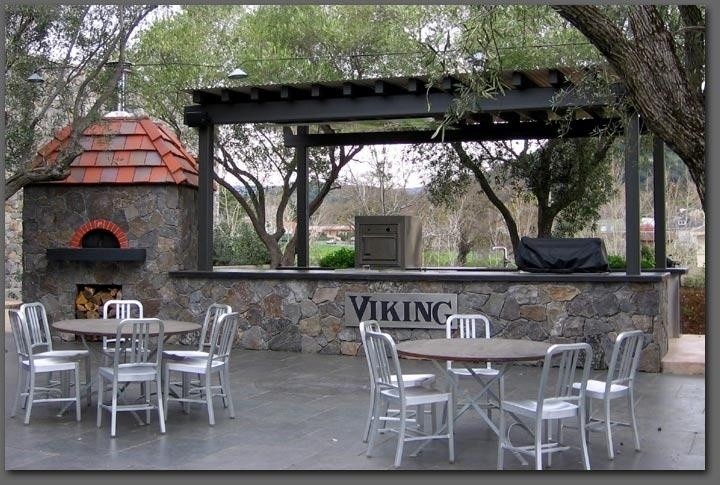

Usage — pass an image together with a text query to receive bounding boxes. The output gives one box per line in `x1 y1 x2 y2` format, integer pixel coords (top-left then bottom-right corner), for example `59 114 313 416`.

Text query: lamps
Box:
228 68 248 79
468 51 488 62
27 71 44 82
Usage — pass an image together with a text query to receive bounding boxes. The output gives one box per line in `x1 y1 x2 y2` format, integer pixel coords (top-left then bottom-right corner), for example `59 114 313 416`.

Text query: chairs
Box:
8 300 239 439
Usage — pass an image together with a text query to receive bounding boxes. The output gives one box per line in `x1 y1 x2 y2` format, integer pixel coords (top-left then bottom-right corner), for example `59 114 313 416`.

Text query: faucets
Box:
491 245 511 268
